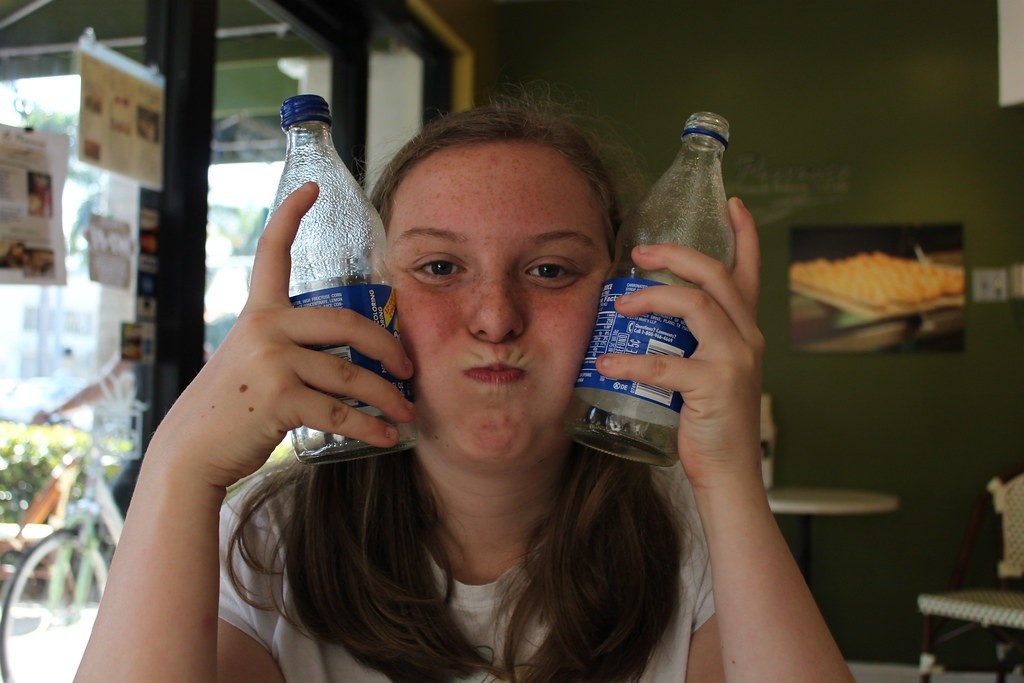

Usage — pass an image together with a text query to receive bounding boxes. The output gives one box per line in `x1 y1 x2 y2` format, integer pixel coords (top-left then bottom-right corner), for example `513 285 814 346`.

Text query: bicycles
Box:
0 392 122 683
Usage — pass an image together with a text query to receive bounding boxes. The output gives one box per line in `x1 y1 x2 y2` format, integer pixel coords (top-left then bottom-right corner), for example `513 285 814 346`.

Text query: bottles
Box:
759 394 777 495
562 111 738 469
267 93 419 464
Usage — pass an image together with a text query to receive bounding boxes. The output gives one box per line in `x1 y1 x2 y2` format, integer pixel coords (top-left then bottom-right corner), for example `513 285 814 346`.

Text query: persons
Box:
31 341 210 518
75 111 853 683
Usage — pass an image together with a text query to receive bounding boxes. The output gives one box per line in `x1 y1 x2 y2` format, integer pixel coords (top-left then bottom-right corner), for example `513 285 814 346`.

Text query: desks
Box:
766 487 902 585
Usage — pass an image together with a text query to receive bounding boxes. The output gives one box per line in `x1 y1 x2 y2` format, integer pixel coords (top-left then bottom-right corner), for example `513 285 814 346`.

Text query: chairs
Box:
917 474 1024 683
